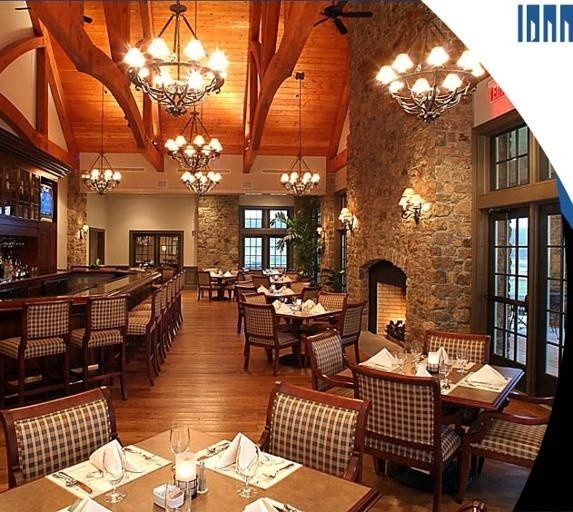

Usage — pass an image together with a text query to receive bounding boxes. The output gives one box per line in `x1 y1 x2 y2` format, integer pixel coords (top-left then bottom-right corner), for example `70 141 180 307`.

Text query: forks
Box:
263 463 295 478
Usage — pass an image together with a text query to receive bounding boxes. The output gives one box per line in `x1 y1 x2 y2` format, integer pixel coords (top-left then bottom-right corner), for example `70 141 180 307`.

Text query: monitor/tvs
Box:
39 184 55 220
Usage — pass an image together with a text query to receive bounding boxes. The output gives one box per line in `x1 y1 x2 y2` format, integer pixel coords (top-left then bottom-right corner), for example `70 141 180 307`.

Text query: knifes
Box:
59 472 94 493
197 442 231 461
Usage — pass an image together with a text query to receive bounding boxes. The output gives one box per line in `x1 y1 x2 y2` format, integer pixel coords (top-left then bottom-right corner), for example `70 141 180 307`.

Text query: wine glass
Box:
235 435 260 498
165 481 191 512
169 426 190 469
439 357 453 390
103 449 127 502
277 296 317 316
395 349 408 374
458 347 470 373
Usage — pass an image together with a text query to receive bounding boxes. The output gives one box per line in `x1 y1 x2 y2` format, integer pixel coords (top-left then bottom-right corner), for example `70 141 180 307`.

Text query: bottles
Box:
196 462 209 495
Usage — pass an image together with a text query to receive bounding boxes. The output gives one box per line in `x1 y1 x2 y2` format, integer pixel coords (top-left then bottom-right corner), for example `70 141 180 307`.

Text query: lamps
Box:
399 187 431 225
279 73 320 198
338 208 356 234
165 107 223 173
79 81 121 198
376 19 483 124
122 0 226 118
179 170 220 198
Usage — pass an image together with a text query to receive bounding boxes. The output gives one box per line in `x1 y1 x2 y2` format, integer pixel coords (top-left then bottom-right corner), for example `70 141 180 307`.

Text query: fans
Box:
314 0 374 34
